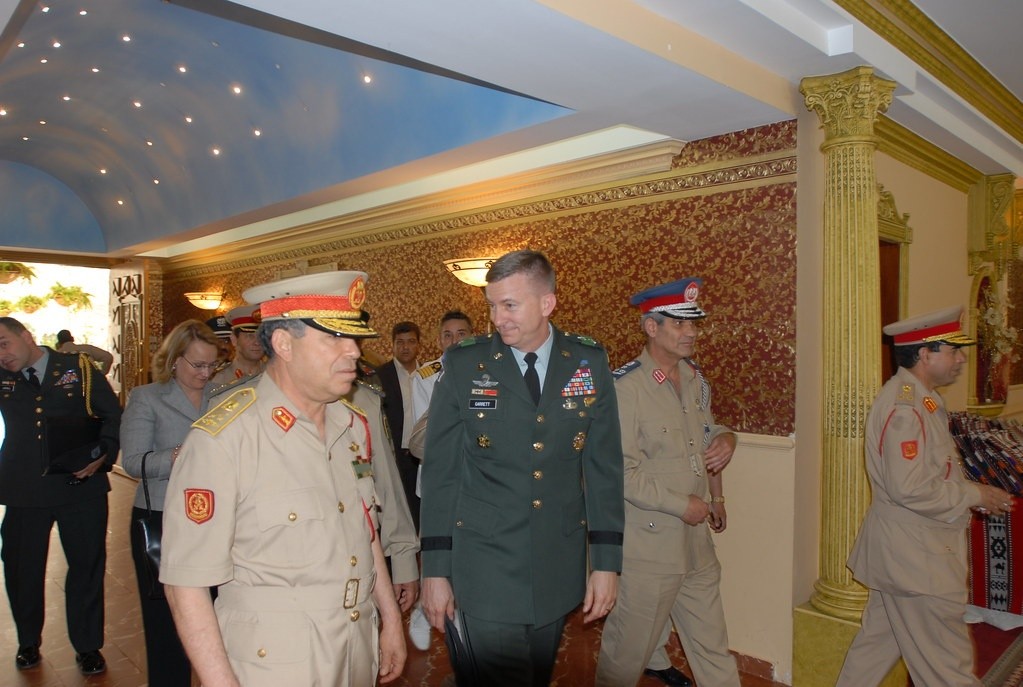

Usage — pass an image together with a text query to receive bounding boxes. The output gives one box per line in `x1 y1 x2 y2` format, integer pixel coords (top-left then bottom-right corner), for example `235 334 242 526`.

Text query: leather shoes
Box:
644 666 692 687
17 645 41 669
76 648 106 674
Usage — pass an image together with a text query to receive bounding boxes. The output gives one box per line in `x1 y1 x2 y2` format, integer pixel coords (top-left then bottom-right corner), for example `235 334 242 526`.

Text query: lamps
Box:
184 292 223 318
442 256 499 337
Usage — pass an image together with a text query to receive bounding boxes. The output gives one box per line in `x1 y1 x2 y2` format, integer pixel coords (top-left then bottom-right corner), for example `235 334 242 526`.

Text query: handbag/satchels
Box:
42 414 104 473
137 450 165 599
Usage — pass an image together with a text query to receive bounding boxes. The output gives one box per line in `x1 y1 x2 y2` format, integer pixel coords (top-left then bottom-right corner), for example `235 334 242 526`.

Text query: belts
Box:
399 449 413 456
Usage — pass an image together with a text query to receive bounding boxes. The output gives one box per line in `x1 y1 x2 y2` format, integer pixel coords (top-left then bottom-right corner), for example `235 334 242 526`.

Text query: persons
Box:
418 250 625 687
55 330 113 375
594 276 742 687
158 271 407 687
835 306 1013 687
206 304 475 649
120 319 230 687
0 317 122 674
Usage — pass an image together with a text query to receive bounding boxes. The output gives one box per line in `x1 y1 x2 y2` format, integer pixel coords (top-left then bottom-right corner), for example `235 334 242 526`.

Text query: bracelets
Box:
712 496 724 503
174 443 182 459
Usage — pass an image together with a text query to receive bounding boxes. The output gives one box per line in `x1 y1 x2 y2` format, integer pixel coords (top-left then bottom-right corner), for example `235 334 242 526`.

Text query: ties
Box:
523 352 541 407
26 368 40 392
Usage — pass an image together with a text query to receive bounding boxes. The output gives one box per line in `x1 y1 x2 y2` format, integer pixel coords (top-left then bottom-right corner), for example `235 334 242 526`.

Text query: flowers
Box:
976 284 1023 362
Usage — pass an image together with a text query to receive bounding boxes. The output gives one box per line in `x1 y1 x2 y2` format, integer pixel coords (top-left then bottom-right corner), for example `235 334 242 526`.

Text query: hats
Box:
630 278 708 320
883 305 977 346
445 608 482 687
53 441 103 471
205 316 233 338
242 270 377 338
358 310 370 324
225 303 261 332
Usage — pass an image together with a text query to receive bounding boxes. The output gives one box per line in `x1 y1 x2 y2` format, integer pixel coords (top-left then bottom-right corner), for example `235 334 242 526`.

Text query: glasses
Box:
180 354 219 369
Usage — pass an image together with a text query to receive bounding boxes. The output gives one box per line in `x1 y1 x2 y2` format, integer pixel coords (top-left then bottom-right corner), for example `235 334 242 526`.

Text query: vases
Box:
983 355 1008 405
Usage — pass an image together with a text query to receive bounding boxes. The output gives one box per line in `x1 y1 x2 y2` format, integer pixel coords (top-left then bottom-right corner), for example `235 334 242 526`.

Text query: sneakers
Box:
406 606 432 651
